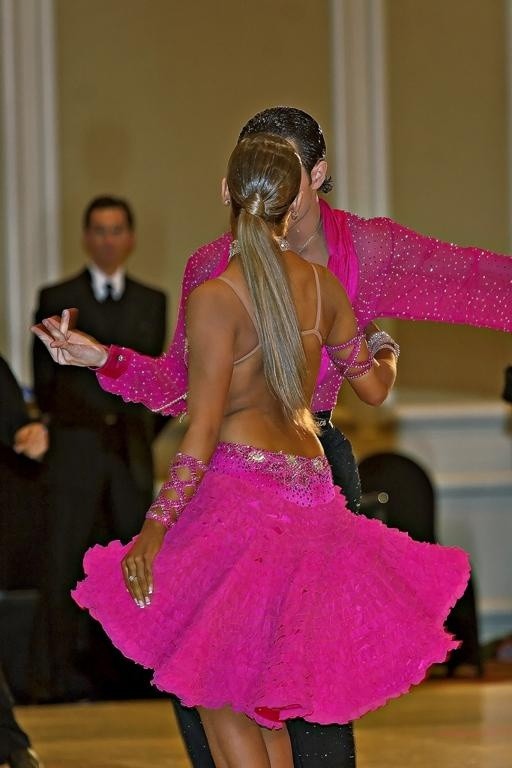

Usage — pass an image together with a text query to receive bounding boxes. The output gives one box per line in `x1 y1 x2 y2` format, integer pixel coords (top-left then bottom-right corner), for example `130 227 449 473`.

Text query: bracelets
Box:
143 450 207 533
366 331 399 359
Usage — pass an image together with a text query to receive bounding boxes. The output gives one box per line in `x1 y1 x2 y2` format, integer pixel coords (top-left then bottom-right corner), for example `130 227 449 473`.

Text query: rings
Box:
126 574 137 582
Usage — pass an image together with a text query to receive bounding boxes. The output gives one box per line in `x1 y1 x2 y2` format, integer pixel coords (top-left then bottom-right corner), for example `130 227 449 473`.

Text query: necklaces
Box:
227 235 290 256
294 214 324 255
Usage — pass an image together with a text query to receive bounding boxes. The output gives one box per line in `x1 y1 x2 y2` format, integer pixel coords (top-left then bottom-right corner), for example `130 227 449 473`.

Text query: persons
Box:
28 192 169 704
69 132 470 767
0 361 52 705
27 104 512 767
